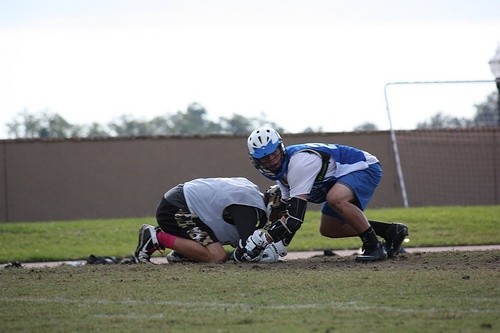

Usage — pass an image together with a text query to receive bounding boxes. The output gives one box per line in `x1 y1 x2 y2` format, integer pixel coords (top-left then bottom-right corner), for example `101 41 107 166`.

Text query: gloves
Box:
259 238 288 263
232 228 272 263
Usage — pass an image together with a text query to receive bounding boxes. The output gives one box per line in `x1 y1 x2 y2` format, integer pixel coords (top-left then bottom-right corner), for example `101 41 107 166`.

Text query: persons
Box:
241 127 409 262
135 176 294 264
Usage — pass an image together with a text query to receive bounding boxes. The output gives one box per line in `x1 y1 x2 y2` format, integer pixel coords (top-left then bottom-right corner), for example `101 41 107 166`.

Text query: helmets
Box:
246 126 288 181
264 184 285 221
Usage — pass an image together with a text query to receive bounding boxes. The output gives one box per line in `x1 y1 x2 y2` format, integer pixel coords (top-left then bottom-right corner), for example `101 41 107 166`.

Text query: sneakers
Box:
355 240 387 263
383 221 408 257
167 249 189 265
135 225 165 265
